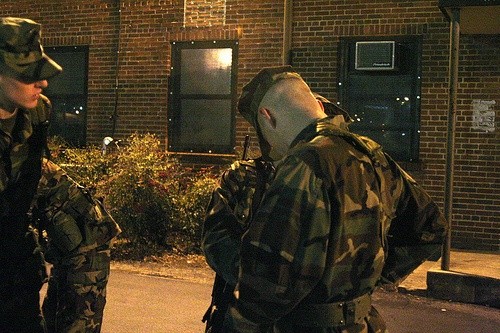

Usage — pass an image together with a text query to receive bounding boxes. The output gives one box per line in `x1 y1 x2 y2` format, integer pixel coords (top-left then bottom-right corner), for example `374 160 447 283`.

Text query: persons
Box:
220 64 448 333
0 16 61 333
202 94 354 332
29 156 123 333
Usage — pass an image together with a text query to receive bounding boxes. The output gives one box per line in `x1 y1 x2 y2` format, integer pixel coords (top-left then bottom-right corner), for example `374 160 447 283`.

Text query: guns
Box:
202 135 251 333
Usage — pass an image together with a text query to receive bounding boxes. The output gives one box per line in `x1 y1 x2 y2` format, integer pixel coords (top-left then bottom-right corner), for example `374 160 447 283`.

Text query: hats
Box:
0 16 63 84
237 65 301 161
